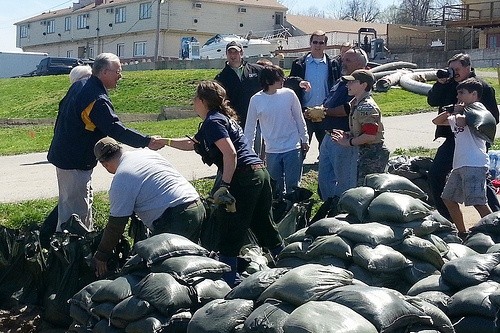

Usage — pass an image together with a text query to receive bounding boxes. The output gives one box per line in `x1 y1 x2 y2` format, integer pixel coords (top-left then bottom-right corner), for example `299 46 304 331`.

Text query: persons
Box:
304 48 369 204
153 80 285 256
427 53 499 224
214 41 267 162
92 136 206 277
286 30 340 162
431 77 493 233
69 64 94 85
243 64 310 195
47 52 165 233
331 69 390 187
329 42 353 66
256 59 273 67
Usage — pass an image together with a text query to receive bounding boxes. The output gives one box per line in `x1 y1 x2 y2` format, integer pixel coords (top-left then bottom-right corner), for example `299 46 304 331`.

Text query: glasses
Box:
352 45 357 53
104 67 122 74
312 40 325 45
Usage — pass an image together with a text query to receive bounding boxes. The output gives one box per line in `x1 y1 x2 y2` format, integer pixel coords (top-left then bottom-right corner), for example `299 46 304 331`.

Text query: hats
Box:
226 41 243 52
341 69 376 87
94 137 118 160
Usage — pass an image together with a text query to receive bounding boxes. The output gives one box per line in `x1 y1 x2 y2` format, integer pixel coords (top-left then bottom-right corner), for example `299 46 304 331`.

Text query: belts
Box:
237 164 265 172
325 129 333 134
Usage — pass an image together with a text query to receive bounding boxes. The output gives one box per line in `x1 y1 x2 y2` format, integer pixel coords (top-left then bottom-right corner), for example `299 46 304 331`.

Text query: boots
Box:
221 257 237 286
268 241 286 267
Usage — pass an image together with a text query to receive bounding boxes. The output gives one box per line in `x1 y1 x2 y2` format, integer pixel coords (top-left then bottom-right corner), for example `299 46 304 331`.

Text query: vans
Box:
35 58 93 77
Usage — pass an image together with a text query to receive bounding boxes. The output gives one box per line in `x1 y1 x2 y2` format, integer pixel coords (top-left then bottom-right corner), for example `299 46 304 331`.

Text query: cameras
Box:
436 67 455 79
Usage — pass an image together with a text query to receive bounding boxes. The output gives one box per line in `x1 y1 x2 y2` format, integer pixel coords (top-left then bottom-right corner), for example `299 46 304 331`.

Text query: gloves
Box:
214 187 228 206
226 192 236 213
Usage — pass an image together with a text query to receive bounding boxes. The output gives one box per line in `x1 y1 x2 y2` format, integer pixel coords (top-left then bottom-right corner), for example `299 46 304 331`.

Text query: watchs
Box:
167 138 172 146
220 180 230 187
349 138 353 146
